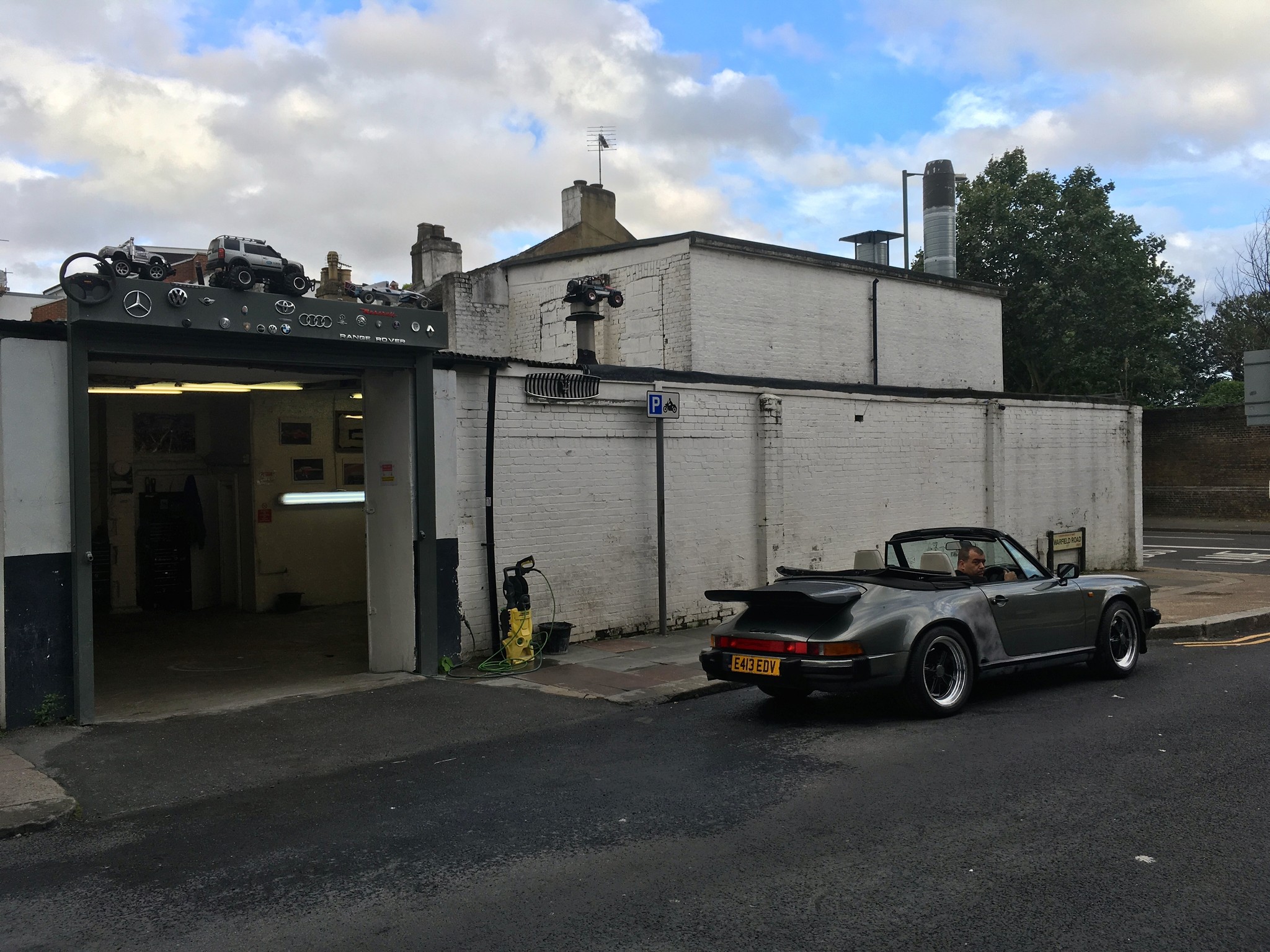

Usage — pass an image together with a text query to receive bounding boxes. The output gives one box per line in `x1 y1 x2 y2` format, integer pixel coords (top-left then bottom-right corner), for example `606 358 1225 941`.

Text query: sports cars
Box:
698 526 1163 718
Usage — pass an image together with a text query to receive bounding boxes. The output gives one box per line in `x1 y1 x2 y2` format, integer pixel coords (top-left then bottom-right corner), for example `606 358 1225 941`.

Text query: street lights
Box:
901 169 969 270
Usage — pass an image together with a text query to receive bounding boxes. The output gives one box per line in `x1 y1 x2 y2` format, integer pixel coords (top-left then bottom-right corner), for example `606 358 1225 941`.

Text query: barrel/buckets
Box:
538 622 576 655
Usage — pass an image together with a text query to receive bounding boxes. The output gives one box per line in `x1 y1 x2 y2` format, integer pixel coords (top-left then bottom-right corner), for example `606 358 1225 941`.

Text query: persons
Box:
955 545 1018 582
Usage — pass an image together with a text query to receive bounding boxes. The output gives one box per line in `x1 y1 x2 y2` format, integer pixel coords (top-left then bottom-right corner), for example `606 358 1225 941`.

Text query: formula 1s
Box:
345 280 432 311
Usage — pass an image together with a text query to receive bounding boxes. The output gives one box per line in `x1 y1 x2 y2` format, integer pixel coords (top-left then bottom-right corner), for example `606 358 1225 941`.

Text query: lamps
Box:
999 403 1006 411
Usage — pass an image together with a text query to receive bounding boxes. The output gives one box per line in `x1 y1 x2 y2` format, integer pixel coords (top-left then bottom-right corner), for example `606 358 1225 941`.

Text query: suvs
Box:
204 235 317 296
565 275 624 308
97 238 176 280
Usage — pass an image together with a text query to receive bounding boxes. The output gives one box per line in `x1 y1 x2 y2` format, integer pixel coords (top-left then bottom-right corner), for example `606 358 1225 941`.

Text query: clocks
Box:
109 460 134 493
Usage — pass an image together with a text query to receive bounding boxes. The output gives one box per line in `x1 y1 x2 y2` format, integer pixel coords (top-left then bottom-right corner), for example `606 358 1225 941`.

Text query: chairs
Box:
919 551 957 576
853 550 886 569
